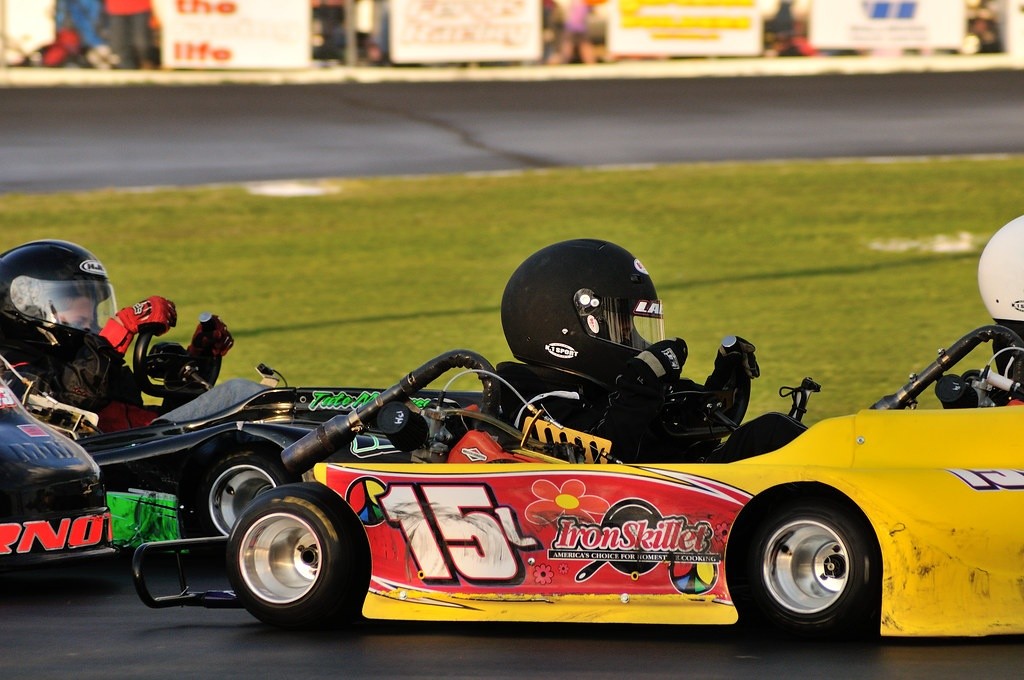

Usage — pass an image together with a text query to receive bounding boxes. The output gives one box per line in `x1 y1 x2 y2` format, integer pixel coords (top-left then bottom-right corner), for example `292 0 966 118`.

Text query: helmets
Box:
502 238 663 390
0 240 117 408
977 212 1023 322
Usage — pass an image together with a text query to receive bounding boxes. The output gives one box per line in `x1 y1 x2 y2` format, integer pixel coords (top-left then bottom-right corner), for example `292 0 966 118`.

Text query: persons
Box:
311 0 388 67
495 238 760 464
541 0 606 65
968 10 1000 54
975 216 1023 405
1 239 234 441
764 31 815 57
28 1 159 69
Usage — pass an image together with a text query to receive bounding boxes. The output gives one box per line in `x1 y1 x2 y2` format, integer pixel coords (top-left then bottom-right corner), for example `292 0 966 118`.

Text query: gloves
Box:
623 337 687 390
706 336 760 397
115 296 177 337
187 314 234 362
